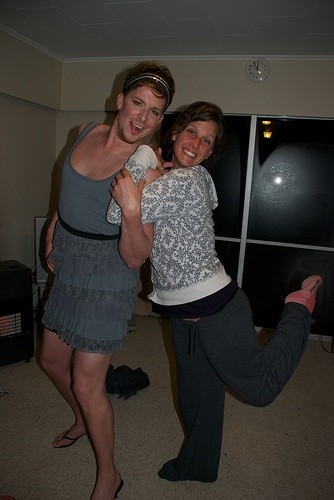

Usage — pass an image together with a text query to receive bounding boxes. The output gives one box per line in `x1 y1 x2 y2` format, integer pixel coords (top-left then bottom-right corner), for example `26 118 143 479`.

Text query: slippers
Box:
88 478 125 500
52 425 91 449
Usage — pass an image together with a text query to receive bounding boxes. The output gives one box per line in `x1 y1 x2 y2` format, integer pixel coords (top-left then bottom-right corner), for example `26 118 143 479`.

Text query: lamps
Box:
263 129 273 139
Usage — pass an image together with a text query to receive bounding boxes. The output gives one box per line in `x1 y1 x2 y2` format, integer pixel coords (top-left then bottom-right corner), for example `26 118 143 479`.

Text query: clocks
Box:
245 57 271 83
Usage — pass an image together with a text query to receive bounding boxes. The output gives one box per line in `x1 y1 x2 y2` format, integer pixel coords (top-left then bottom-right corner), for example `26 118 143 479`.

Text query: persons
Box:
38 63 176 500
104 102 323 483
